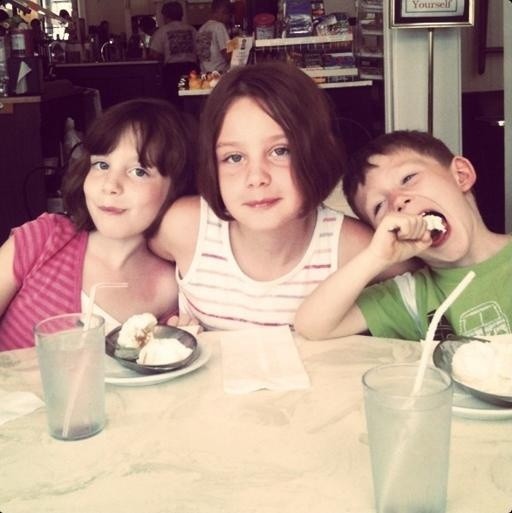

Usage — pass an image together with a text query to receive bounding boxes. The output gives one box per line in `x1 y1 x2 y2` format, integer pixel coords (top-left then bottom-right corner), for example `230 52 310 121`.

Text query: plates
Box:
105 323 197 375
419 339 512 423
432 333 512 410
104 331 210 387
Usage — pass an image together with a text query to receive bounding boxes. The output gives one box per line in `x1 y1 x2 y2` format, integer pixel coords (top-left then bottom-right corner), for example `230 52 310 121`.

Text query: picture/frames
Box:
389 0 475 30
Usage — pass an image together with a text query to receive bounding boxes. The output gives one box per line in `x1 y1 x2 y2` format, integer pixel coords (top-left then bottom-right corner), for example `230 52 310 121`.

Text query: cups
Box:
33 311 107 441
361 361 455 513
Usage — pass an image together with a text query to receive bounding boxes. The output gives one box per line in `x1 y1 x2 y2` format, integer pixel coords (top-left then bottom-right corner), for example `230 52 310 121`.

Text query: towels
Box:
0 388 45 425
220 325 311 394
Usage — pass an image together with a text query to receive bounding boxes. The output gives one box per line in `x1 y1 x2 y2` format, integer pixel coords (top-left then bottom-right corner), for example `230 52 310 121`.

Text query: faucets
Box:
138 39 148 61
100 37 116 62
48 41 64 67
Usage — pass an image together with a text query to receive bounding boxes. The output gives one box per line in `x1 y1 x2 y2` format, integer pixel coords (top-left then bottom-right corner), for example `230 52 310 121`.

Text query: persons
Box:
293 128 512 344
145 58 422 336
25 0 234 114
0 96 195 353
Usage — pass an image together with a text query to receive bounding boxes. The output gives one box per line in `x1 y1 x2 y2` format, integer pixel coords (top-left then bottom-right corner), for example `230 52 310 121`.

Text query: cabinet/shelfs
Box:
354 0 384 80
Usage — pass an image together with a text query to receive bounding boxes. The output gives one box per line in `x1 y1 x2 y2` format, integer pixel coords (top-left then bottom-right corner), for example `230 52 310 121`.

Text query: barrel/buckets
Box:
253 13 276 40
